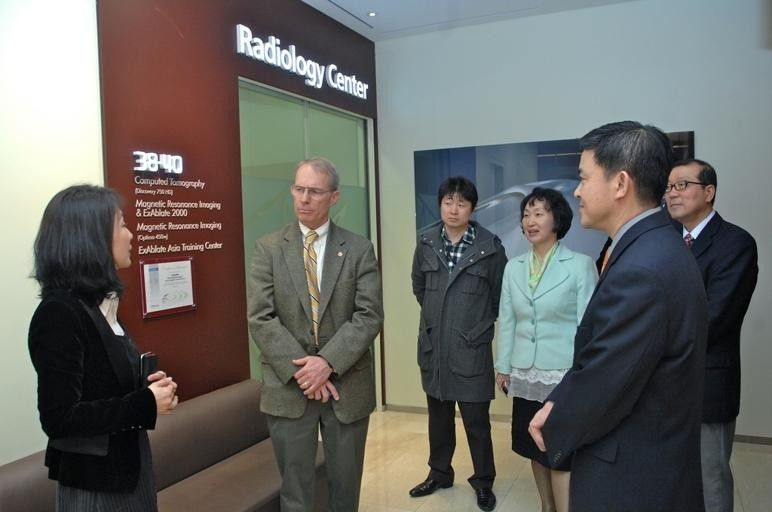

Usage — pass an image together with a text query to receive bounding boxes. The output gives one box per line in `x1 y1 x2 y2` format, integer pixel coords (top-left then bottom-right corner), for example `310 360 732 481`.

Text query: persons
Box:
495 186 600 512
665 159 759 512
528 120 706 512
242 155 385 512
410 174 509 512
26 182 178 512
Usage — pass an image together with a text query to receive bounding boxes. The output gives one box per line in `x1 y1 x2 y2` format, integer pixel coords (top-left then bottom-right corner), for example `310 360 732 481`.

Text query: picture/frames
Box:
139 256 199 322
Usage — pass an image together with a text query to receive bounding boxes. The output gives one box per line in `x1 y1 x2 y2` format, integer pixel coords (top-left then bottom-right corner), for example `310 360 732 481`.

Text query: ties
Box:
680 235 694 251
300 232 323 347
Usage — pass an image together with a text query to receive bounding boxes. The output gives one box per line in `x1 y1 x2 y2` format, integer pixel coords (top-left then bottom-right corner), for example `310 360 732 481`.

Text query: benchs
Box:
0 379 328 512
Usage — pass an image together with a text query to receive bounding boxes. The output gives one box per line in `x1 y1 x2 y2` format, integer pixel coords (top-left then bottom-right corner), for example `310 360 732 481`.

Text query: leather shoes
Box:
409 477 455 498
477 487 498 512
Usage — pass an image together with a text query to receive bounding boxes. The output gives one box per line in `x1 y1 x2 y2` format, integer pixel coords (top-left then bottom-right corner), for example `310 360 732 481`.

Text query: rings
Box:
304 382 309 387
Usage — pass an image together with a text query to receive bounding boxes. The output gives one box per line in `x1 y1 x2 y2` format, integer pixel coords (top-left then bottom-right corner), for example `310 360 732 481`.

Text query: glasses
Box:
666 179 707 192
290 184 330 197
